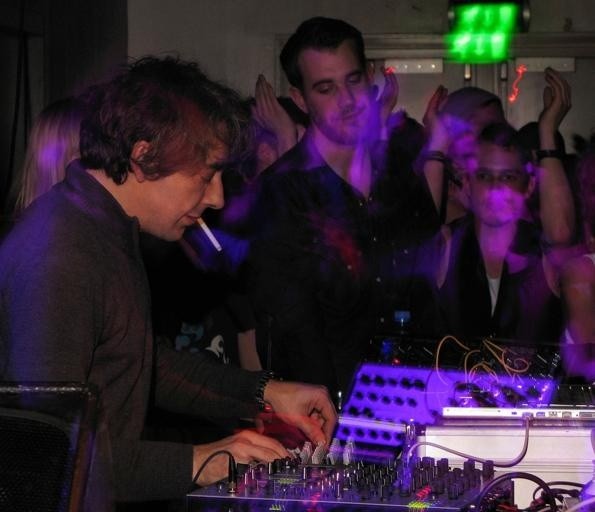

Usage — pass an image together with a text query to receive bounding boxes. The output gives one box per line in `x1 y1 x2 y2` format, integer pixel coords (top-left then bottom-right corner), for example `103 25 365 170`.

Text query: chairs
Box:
0 381 101 512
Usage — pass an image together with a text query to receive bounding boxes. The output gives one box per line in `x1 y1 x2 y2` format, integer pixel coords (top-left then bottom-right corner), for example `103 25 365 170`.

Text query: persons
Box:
1 54 337 512
4 99 86 237
153 17 594 394
